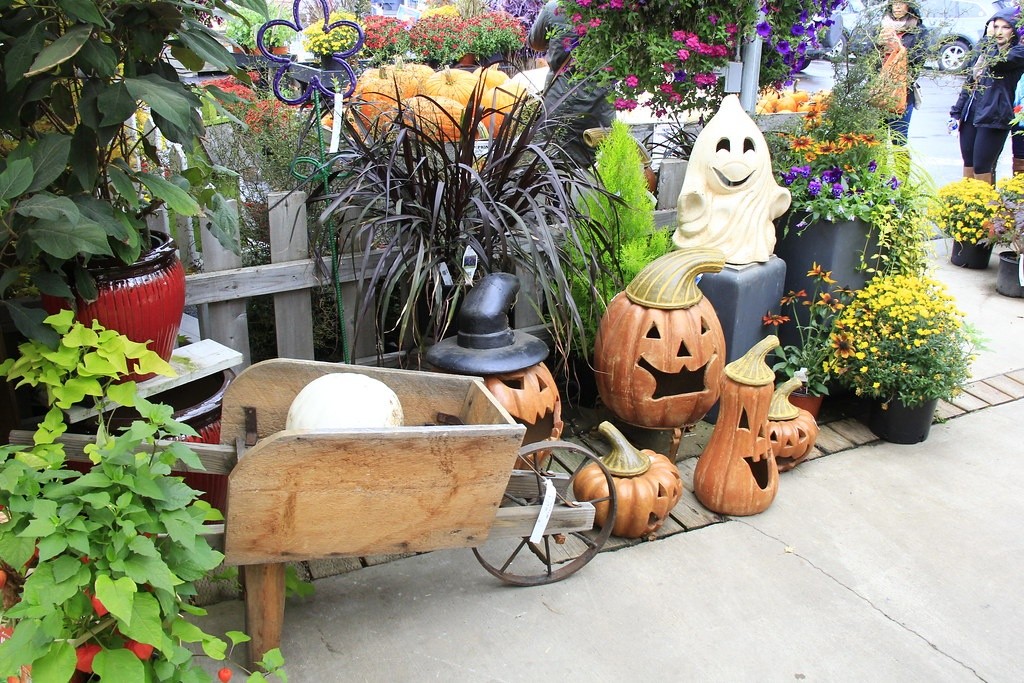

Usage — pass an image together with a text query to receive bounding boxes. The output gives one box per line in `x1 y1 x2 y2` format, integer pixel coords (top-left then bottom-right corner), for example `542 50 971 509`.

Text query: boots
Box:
974 173 994 192
963 166 973 181
1012 157 1024 177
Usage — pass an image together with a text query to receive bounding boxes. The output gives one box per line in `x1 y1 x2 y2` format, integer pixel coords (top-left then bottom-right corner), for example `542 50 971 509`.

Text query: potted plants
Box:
257 58 675 416
0 0 271 385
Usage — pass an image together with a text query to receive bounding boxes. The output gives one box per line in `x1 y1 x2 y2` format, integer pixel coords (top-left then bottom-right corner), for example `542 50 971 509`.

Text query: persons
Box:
863 0 930 147
946 7 1024 186
1011 73 1024 178
525 0 616 168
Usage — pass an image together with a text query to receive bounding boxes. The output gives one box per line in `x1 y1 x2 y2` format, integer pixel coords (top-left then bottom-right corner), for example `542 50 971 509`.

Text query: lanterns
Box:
766 377 817 472
593 246 726 428
573 421 682 537
426 273 563 470
694 335 779 516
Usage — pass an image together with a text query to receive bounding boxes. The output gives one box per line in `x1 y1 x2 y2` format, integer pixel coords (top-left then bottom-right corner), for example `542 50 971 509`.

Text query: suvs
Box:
823 1 1001 72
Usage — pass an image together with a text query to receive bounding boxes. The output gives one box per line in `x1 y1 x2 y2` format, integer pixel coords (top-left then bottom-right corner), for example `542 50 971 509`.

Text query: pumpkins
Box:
753 78 831 112
286 372 404 432
324 54 550 142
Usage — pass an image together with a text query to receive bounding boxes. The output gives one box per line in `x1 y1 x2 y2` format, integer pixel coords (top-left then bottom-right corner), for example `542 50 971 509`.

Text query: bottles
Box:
948 119 959 136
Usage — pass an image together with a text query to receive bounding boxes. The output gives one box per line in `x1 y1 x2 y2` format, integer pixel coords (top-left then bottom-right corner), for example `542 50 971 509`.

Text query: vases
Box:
233 45 246 54
788 392 825 417
697 255 787 425
867 393 939 445
996 251 1024 298
85 368 235 526
429 58 442 69
457 53 474 67
271 46 287 55
952 239 994 269
321 53 345 70
773 207 885 357
253 47 269 57
478 48 503 67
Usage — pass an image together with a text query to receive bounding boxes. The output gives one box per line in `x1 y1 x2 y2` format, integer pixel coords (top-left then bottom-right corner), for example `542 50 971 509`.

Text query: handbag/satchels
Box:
911 81 923 110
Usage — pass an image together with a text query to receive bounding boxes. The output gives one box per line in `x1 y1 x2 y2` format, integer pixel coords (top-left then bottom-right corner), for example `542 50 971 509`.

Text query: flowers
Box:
546 0 1024 410
141 0 529 180
0 308 315 683
